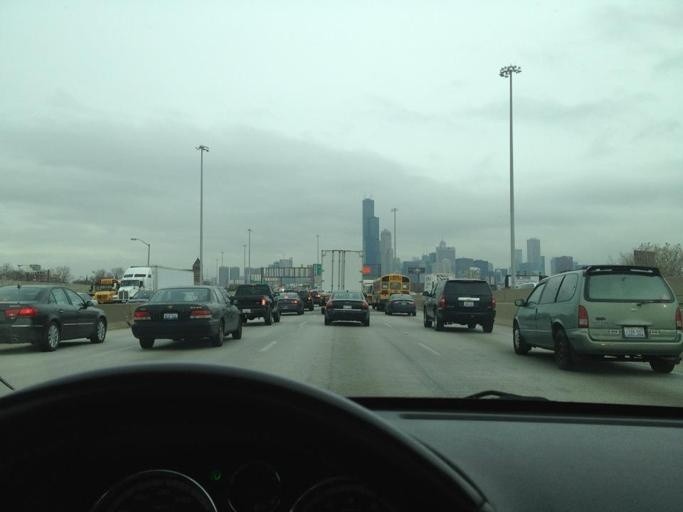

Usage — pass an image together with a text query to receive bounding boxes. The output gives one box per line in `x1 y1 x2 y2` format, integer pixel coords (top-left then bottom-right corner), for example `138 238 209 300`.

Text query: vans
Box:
512 265 682 372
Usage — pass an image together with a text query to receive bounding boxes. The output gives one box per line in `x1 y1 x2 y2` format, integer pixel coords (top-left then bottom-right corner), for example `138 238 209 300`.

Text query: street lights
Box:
247 228 251 282
389 208 397 274
499 65 522 288
242 243 247 284
220 250 223 285
130 238 150 267
194 145 209 287
315 234 319 264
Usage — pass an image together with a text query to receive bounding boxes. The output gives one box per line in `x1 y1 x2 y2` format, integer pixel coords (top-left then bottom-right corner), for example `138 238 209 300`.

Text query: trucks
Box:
422 272 456 298
320 249 364 314
114 267 195 305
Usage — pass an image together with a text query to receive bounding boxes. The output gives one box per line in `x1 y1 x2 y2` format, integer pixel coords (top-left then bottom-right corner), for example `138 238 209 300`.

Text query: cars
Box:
324 289 371 326
0 285 107 351
517 281 540 289
131 286 243 349
271 273 414 315
125 289 154 305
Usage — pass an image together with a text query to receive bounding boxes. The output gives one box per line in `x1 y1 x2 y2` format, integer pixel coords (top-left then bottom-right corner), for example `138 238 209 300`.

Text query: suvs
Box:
423 277 496 330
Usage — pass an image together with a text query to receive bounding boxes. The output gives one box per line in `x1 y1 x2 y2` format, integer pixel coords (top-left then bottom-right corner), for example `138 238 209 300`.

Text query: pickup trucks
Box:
230 282 282 325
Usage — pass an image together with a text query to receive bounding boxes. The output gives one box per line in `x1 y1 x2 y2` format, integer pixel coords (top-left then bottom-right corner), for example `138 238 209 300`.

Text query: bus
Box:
91 278 122 304
